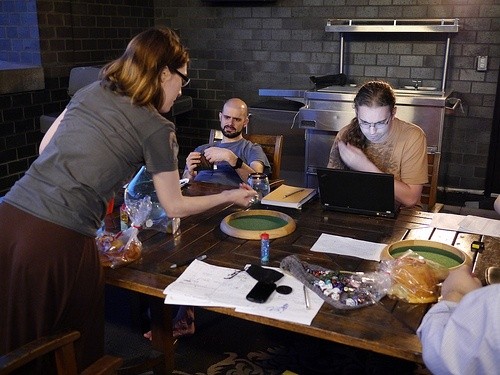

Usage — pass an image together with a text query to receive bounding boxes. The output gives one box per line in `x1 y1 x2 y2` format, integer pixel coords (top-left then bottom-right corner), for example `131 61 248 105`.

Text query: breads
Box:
97 233 141 267
386 261 440 304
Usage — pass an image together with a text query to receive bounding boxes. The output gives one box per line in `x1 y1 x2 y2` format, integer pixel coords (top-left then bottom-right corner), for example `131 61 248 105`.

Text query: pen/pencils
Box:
303 285 309 309
170 254 208 269
283 188 305 198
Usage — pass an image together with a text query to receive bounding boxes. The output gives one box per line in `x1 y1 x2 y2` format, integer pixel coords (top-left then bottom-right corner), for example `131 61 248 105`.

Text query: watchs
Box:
232 157 243 170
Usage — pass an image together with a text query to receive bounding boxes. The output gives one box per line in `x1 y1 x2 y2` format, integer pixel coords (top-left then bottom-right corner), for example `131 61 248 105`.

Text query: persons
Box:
181 97 272 188
416 264 500 375
326 81 429 211
494 195 500 214
0 25 257 375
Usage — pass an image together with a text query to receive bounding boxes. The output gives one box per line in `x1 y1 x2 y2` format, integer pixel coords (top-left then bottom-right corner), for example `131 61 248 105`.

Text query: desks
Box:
40 96 192 134
98 169 500 375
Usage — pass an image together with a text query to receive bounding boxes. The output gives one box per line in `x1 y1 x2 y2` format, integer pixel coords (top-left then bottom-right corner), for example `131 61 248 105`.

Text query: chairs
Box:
421 151 444 213
209 128 284 179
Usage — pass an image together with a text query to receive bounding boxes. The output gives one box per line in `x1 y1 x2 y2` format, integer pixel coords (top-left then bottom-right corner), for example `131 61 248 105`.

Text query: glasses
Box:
167 64 190 87
357 108 394 129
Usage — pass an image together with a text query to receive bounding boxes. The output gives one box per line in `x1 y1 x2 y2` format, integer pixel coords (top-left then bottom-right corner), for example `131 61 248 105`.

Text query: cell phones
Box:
246 281 276 304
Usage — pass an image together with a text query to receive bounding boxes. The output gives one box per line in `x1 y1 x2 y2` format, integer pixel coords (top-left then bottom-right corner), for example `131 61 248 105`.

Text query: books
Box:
260 184 317 210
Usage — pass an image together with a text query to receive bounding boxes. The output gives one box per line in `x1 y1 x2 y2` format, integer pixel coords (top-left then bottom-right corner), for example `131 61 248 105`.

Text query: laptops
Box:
318 172 401 217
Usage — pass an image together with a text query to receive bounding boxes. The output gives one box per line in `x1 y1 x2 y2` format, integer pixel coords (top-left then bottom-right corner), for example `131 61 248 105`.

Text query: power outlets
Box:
476 55 488 72
